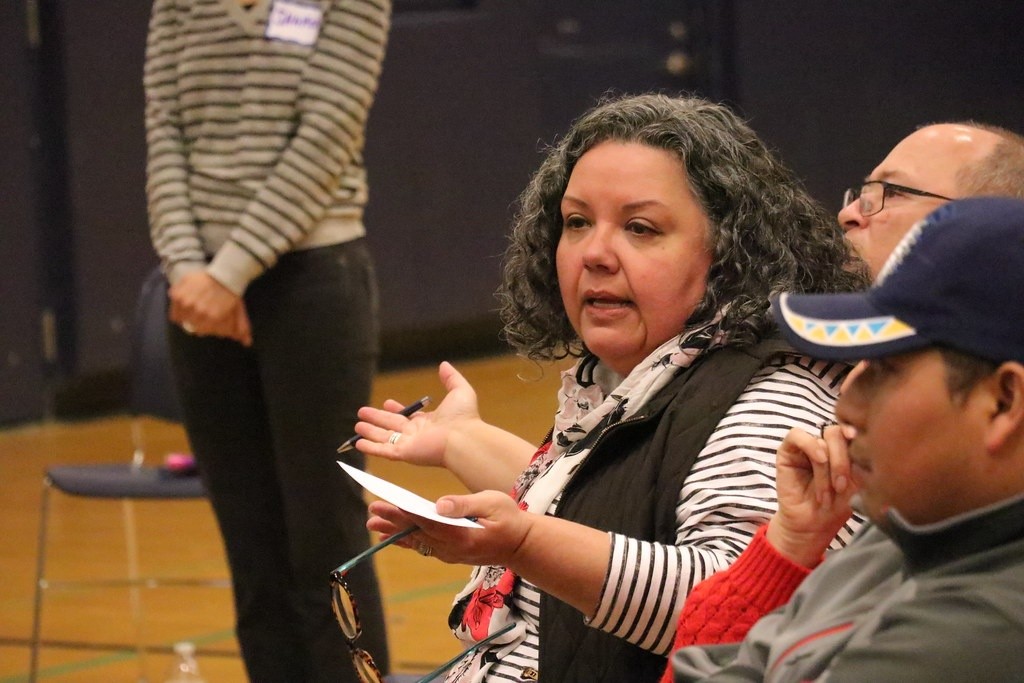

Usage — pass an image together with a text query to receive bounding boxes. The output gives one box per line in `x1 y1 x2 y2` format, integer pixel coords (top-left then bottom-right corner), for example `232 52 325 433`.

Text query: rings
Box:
183 323 195 335
416 543 432 556
821 422 837 438
389 432 401 444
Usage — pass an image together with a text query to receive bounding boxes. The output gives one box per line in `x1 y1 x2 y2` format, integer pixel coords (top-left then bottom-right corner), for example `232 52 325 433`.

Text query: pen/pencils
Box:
337 396 432 454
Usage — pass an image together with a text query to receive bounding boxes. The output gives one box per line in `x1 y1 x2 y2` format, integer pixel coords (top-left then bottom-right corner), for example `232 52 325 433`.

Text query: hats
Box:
771 197 1023 363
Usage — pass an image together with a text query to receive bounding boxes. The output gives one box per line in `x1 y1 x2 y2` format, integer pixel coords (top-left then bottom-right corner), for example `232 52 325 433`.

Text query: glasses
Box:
328 515 517 683
843 181 953 218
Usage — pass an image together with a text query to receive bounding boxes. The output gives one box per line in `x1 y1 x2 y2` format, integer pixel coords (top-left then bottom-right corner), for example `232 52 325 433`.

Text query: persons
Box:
838 121 1024 285
671 197 1024 683
354 96 870 683
143 0 391 683
660 424 859 683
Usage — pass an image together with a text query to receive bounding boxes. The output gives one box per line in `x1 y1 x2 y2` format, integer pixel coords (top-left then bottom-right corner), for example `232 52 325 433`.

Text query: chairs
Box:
28 263 207 683
